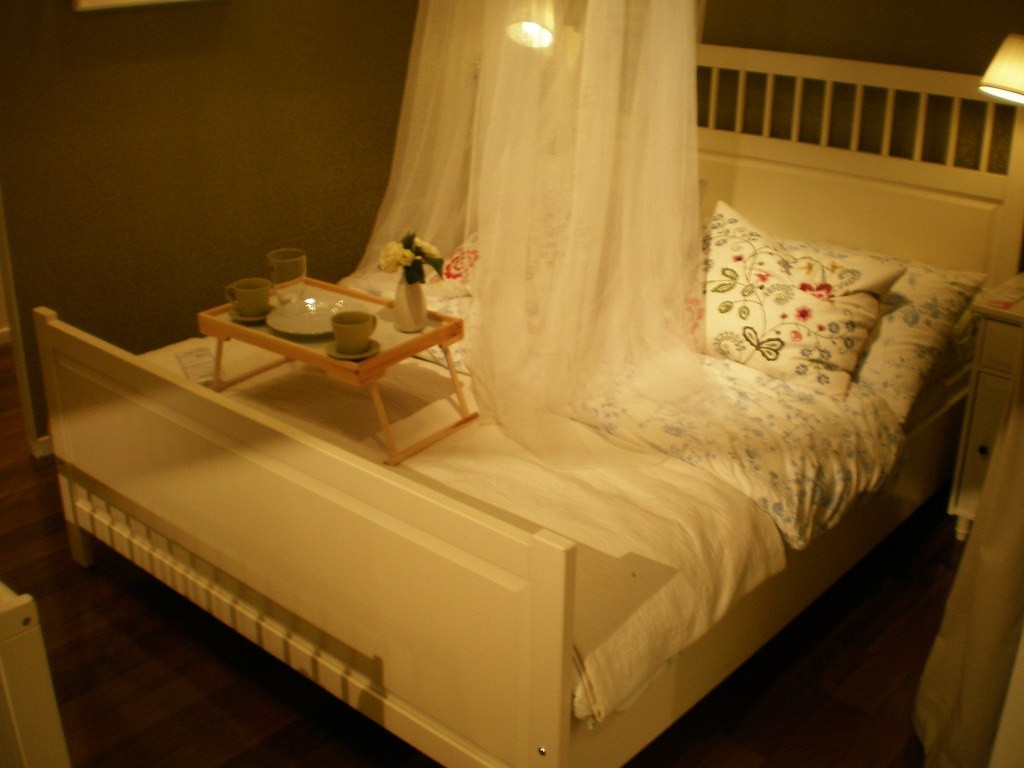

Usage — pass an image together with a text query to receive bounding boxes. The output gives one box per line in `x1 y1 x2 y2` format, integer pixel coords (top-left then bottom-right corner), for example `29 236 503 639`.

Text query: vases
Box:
394 269 427 333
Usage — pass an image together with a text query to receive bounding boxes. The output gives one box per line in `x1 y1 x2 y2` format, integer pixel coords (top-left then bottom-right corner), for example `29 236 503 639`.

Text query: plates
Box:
226 304 278 323
266 301 341 336
328 338 380 360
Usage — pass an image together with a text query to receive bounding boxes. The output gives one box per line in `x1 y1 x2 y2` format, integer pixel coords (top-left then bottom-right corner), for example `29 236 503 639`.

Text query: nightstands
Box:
946 273 1024 541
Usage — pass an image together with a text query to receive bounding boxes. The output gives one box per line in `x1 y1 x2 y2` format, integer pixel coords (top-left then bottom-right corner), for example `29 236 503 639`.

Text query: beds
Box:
32 42 1024 768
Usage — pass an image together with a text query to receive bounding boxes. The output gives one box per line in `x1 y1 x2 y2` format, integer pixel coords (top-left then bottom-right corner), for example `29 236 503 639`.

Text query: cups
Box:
224 278 271 316
267 248 306 318
331 312 377 354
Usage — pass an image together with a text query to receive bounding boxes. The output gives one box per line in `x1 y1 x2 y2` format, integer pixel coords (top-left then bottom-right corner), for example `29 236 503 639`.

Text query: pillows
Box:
856 252 985 425
664 201 907 398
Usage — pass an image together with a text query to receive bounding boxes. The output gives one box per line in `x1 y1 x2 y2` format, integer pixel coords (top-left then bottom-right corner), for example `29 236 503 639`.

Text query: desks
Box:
199 276 479 466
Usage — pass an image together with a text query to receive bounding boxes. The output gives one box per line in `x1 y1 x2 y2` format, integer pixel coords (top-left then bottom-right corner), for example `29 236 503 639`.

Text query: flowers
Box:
377 232 445 284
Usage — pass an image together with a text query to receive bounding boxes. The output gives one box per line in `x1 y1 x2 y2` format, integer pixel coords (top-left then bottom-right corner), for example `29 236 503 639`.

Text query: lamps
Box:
978 33 1024 106
504 0 556 48
